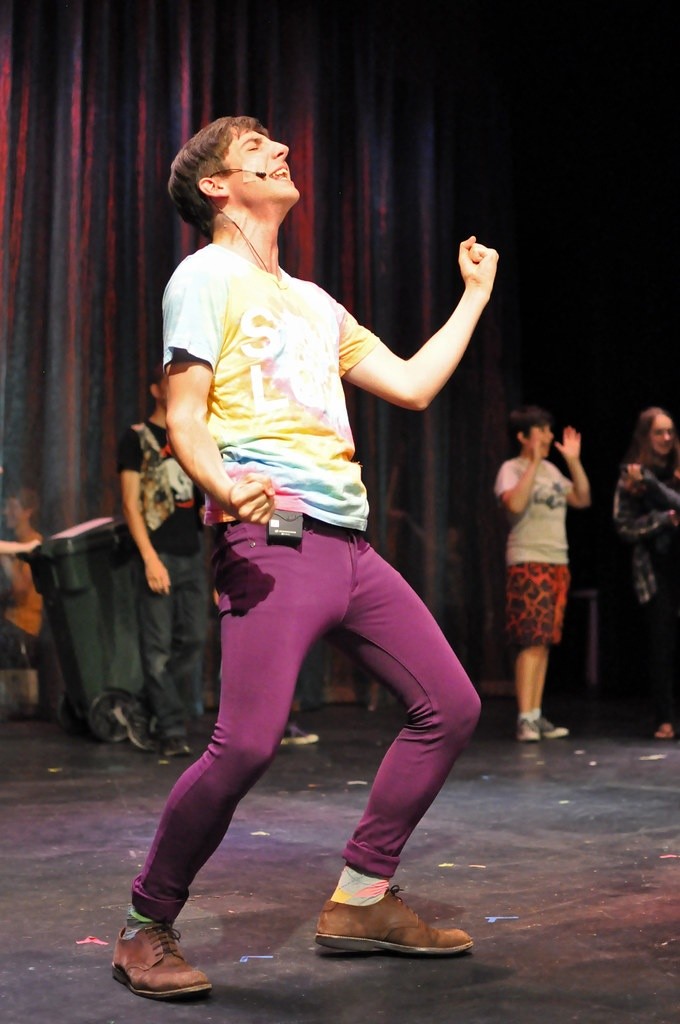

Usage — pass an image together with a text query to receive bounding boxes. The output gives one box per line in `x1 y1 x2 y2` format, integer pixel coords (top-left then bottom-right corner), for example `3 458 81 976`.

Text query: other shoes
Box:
653 724 674 740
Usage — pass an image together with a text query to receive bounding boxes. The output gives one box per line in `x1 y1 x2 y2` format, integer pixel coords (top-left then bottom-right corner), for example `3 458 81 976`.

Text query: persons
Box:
113 118 498 998
0 467 46 667
493 406 590 739
614 409 679 741
119 355 213 760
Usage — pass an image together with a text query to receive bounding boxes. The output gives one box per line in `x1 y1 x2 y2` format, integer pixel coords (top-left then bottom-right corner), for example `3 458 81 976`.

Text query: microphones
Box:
208 168 267 178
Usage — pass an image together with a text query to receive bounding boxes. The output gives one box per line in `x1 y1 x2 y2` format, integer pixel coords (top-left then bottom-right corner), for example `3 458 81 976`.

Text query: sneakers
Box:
112 700 158 752
537 715 570 738
516 715 541 743
279 722 320 744
162 736 193 758
111 924 212 997
314 884 473 955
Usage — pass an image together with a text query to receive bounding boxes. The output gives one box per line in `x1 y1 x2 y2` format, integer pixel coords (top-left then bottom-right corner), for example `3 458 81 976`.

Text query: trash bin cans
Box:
15 513 157 753
